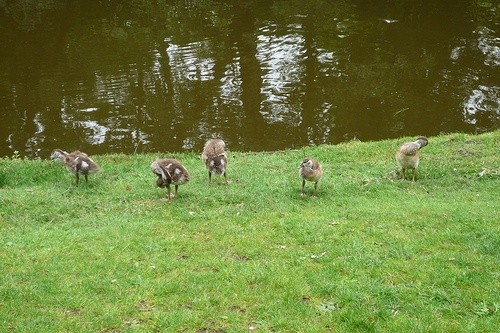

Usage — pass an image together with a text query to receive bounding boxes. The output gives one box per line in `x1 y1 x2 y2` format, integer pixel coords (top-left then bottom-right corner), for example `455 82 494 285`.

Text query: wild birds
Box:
201 138 228 184
395 137 428 182
297 157 323 198
50 148 101 187
150 158 191 201
